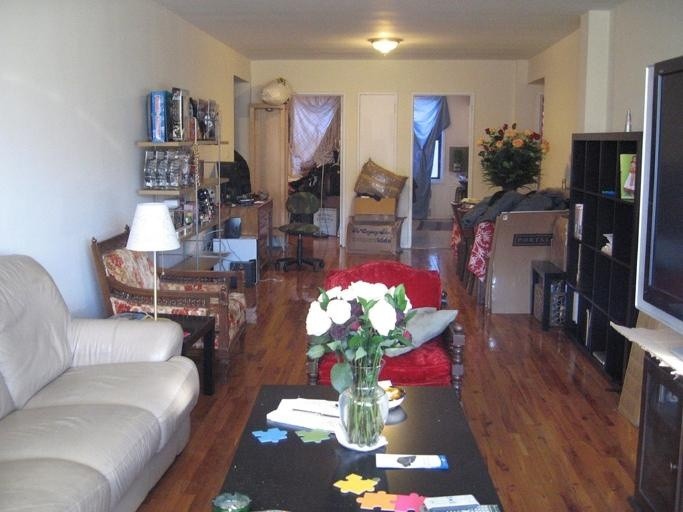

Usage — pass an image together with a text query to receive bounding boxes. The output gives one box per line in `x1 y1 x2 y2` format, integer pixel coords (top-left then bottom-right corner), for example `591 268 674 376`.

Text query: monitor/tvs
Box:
216 161 244 204
635 55 683 361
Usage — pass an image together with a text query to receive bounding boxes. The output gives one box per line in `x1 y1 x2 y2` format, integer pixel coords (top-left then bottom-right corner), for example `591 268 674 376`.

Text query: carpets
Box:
412 230 453 250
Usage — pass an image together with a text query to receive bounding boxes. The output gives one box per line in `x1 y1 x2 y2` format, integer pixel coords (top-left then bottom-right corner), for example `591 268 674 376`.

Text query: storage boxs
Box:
311 207 338 238
550 216 569 273
344 156 409 259
533 282 565 328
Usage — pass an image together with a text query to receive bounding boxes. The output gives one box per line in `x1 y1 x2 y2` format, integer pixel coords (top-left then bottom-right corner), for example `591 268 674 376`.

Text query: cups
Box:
210 490 251 511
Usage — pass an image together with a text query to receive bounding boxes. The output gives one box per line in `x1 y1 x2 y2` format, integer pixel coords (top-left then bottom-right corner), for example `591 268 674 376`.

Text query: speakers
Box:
224 217 241 238
230 261 256 288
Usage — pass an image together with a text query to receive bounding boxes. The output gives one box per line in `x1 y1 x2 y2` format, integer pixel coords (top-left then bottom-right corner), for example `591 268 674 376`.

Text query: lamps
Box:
366 36 406 58
120 201 182 321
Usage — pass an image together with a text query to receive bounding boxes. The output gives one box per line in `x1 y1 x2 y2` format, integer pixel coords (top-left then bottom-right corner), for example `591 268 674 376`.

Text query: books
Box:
572 203 584 240
150 87 221 145
619 153 636 200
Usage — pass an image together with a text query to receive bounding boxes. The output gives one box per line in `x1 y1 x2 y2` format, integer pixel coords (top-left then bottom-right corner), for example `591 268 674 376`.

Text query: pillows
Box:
385 306 459 359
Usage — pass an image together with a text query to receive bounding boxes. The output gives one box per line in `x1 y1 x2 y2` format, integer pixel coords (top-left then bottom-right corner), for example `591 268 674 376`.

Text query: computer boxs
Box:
213 236 260 284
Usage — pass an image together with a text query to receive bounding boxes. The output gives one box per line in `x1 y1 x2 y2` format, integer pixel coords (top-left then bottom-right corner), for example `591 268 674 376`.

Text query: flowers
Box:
475 122 550 192
298 277 418 445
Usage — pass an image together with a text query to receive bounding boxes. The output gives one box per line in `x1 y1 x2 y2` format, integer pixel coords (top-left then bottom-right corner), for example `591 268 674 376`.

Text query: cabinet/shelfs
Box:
622 349 683 511
213 196 274 286
561 128 643 388
133 137 235 281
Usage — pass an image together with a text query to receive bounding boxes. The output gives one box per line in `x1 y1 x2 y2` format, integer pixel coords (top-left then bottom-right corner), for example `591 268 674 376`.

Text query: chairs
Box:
87 223 249 387
445 185 569 315
305 259 468 411
269 190 326 273
289 171 319 193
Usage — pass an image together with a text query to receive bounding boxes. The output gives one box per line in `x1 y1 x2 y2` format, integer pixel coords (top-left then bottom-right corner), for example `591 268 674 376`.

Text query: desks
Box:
108 311 214 400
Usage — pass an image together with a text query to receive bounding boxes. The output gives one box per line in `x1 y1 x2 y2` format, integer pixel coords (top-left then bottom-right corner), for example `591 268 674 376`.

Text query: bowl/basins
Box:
384 407 408 426
349 388 404 410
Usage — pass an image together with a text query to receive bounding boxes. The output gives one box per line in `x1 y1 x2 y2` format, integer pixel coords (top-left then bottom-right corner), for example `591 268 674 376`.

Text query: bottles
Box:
623 107 632 132
143 154 191 187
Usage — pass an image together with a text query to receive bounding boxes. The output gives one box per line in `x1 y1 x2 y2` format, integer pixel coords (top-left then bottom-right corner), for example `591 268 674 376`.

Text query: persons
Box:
625 157 639 194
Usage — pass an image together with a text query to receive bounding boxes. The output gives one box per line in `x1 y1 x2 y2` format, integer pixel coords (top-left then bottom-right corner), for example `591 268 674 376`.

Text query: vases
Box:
335 355 391 447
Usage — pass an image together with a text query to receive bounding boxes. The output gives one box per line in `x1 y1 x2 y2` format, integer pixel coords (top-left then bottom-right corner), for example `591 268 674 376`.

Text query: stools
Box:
525 258 565 333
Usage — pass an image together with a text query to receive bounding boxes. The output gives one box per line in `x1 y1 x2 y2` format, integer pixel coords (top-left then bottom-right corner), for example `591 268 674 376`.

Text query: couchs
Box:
0 251 204 511
